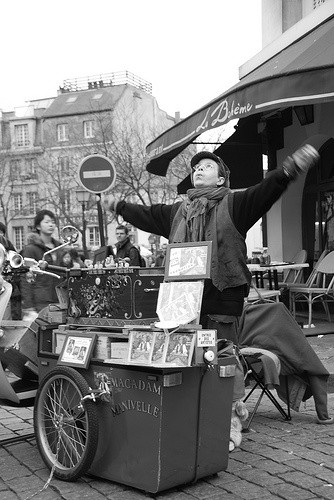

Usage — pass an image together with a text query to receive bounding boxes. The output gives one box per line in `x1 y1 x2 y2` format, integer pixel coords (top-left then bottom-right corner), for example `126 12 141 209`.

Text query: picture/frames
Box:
57 332 97 370
164 240 212 281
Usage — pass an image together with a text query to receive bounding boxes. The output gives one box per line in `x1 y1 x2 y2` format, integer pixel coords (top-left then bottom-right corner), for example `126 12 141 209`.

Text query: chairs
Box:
243 281 281 309
267 250 334 328
207 302 292 433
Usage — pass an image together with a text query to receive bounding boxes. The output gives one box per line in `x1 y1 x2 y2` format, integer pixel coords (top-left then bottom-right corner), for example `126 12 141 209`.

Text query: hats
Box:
190 150 231 179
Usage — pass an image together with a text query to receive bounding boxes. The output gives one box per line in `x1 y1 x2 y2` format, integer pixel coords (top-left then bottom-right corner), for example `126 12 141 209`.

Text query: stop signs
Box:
78 154 116 194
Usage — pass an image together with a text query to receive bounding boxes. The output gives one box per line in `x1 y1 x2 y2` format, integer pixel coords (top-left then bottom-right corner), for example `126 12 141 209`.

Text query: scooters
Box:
0 225 78 407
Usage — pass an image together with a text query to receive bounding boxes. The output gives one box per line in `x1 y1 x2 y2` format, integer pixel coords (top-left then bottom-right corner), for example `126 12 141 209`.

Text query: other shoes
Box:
229 417 242 451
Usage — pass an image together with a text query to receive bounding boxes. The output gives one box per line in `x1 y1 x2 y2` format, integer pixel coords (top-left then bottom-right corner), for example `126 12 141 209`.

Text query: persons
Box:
0 224 16 252
238 299 334 425
108 225 142 266
101 143 321 451
57 243 168 267
21 210 70 268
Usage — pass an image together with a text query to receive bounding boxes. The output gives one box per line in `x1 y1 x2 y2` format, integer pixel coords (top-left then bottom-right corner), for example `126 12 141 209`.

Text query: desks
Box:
246 261 309 290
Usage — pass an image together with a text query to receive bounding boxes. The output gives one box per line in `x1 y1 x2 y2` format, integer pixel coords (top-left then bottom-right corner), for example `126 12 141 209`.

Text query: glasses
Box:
192 164 219 170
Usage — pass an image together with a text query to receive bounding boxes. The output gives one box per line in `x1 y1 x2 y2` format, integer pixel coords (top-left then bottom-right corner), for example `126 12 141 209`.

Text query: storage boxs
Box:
67 266 165 319
